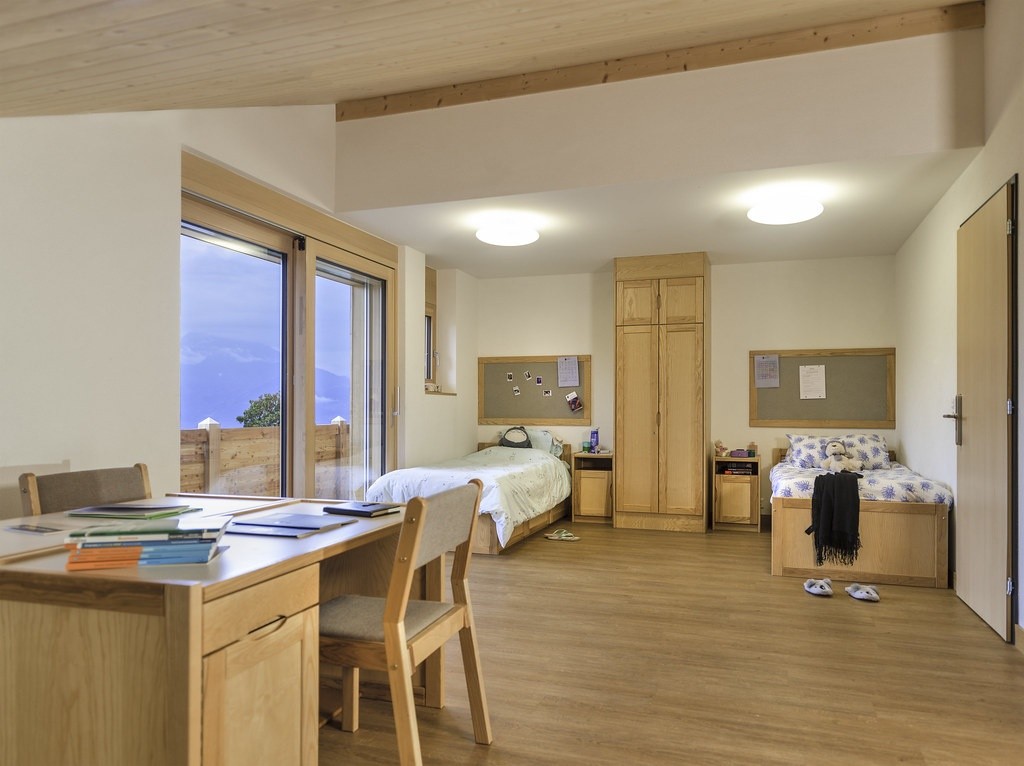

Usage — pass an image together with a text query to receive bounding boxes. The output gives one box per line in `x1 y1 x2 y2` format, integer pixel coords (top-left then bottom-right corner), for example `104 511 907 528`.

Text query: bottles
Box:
590 430 599 452
747 449 755 457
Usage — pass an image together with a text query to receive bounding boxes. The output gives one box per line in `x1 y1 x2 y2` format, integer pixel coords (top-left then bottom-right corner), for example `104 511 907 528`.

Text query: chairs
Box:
319 479 493 766
19 463 152 518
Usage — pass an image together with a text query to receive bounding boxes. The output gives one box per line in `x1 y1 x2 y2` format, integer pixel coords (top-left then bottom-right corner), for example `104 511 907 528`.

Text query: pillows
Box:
784 432 843 468
497 428 564 457
836 433 891 470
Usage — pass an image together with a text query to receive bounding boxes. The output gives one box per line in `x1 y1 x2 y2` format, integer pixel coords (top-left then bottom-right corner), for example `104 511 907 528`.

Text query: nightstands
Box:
571 452 613 523
712 456 761 533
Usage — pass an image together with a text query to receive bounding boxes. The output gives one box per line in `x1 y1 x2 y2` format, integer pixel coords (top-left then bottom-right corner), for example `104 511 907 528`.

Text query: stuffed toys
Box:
499 426 532 448
820 440 865 473
714 438 730 457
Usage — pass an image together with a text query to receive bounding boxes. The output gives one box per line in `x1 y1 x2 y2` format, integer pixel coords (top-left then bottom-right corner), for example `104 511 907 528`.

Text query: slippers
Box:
802 579 833 596
544 529 573 537
548 533 580 540
845 582 880 601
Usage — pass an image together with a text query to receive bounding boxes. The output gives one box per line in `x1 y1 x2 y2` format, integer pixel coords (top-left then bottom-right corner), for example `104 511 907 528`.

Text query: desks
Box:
0 493 445 766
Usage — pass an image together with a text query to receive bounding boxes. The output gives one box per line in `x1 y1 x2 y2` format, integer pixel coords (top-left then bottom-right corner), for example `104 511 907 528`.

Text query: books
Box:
225 522 343 539
725 469 752 475
234 512 357 529
323 501 400 518
67 503 203 521
64 515 235 571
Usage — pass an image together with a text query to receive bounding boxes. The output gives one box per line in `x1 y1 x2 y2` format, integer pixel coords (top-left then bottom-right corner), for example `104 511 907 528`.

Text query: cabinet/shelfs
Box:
613 251 714 533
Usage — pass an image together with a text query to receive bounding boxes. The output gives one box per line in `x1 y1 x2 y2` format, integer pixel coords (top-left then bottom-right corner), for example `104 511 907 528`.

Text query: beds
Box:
388 443 571 555
771 448 949 589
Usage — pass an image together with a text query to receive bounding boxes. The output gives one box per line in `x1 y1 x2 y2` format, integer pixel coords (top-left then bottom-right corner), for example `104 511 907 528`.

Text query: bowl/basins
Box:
425 383 437 391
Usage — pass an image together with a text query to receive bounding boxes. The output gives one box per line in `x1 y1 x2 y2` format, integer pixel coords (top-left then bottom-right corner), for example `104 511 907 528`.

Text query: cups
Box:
583 441 590 452
437 384 442 393
